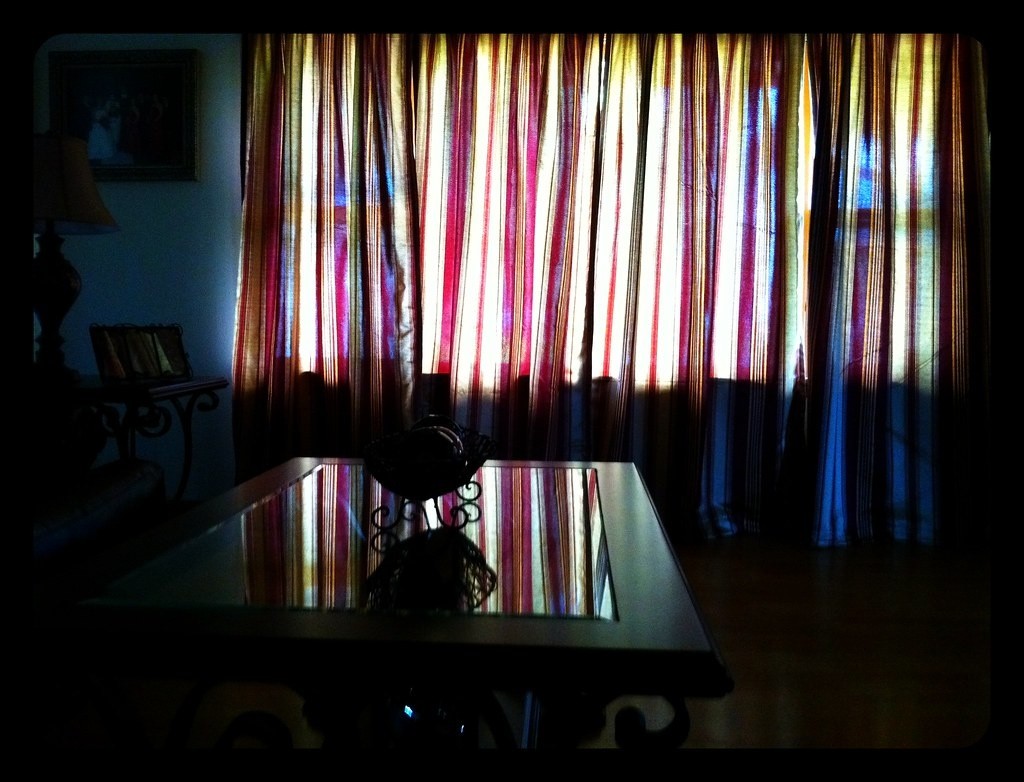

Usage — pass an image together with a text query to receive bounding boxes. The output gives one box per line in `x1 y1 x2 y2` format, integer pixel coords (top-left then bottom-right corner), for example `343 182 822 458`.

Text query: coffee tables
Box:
47 453 736 751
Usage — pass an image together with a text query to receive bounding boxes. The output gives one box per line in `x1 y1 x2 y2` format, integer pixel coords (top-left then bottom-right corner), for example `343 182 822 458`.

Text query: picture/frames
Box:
46 45 206 182
